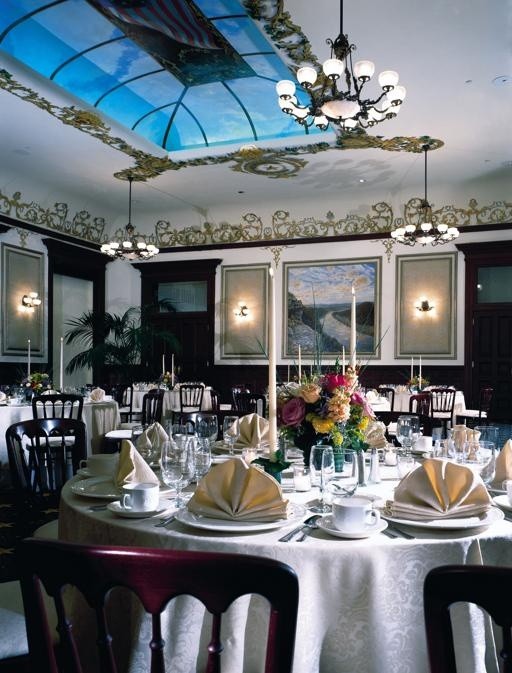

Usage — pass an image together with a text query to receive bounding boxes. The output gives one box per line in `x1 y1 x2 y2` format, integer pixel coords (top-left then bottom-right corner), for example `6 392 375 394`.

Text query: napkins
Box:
112 439 161 498
184 457 288 523
383 457 492 521
494 439 511 487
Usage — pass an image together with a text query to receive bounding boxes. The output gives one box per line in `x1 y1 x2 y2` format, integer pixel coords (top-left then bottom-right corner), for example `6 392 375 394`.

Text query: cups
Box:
331 497 380 531
121 481 159 512
132 424 158 466
321 447 359 498
242 446 258 466
382 414 501 485
14 385 91 406
79 454 119 475
292 463 312 491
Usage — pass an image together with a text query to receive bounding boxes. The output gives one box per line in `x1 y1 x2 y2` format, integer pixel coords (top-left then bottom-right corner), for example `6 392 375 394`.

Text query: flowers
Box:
277 374 374 454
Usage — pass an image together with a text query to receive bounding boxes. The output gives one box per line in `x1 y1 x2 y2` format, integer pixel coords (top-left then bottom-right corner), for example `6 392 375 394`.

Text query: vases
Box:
302 438 346 471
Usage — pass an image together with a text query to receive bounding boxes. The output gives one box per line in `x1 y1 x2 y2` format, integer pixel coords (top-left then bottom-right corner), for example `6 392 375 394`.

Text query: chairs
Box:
7 419 88 532
0 380 509 443
26 394 85 495
423 562 508 673
13 537 297 671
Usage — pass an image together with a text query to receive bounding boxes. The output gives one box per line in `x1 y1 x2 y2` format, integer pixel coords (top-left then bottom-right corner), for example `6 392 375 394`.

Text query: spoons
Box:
279 514 322 542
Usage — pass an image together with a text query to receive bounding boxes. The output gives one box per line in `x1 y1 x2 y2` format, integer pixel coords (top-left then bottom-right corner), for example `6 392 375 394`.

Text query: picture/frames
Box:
280 255 384 363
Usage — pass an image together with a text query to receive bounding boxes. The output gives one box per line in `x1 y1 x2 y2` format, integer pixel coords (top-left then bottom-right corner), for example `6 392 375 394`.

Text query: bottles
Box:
357 446 380 486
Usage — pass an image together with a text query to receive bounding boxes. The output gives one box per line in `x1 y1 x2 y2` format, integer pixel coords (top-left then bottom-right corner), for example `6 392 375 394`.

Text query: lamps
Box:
233 300 252 316
387 143 460 249
272 0 407 130
414 295 435 314
98 171 159 265
21 291 43 306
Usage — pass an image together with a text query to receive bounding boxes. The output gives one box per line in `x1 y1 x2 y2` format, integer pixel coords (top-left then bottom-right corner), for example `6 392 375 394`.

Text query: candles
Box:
171 353 174 387
28 340 31 386
419 354 422 388
349 291 360 371
310 363 312 378
411 356 413 383
288 364 290 384
60 338 64 388
342 346 346 376
162 355 165 376
298 344 302 386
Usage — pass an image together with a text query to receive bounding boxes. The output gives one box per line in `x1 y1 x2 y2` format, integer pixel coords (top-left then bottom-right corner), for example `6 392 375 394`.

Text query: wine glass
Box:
161 412 239 512
307 445 334 514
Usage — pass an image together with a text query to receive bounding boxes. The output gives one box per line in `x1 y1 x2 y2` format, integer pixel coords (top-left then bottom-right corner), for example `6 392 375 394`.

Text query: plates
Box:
379 504 505 529
105 497 173 519
316 515 388 540
174 502 306 532
486 485 512 515
214 439 305 460
77 467 91 478
71 475 176 497
